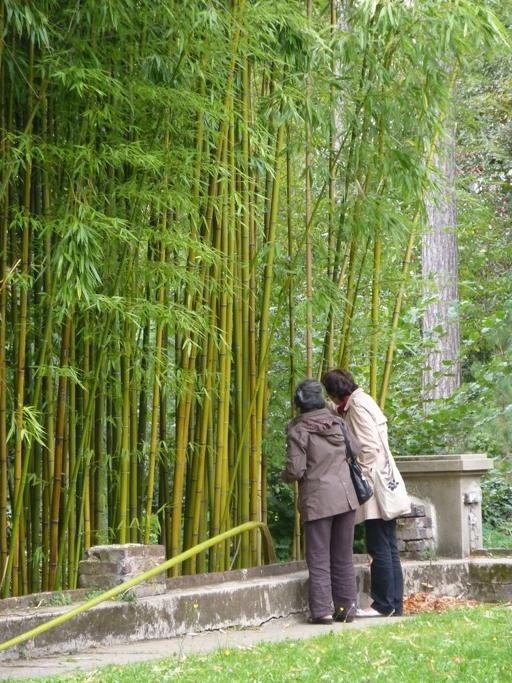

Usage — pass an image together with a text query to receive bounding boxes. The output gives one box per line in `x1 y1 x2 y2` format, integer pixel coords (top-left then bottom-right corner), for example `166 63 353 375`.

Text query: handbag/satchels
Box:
347 456 373 504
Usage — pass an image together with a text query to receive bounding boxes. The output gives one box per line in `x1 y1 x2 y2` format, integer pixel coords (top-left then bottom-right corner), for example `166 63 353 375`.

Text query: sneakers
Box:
308 609 384 624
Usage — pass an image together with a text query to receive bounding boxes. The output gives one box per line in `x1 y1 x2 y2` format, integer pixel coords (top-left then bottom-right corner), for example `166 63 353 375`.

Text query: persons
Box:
322 369 412 618
281 380 360 625
